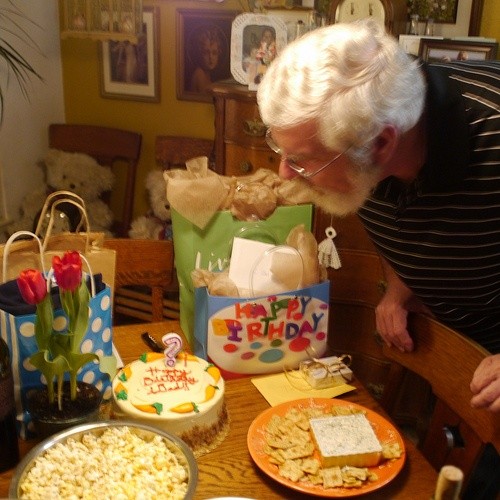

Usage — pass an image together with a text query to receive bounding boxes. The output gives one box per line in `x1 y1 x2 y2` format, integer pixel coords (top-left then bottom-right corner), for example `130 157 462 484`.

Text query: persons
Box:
257 19 500 500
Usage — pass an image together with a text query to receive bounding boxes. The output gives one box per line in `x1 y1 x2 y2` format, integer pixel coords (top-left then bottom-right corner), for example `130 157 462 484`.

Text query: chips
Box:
264 406 401 488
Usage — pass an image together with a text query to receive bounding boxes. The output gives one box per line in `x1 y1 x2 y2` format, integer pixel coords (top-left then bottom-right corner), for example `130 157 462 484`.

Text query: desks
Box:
0 314 451 500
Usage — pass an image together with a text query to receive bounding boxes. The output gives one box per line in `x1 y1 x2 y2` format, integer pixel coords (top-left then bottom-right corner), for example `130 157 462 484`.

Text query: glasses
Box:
266 128 354 180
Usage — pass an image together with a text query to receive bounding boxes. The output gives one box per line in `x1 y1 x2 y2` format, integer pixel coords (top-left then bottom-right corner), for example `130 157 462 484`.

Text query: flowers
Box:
17 253 100 414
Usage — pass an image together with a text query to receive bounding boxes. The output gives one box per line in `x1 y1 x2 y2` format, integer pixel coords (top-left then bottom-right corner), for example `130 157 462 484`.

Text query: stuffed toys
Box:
129 169 183 242
7 148 115 240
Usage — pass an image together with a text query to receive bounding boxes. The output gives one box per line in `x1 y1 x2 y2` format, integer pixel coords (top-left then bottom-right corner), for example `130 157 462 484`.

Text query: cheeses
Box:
308 412 381 467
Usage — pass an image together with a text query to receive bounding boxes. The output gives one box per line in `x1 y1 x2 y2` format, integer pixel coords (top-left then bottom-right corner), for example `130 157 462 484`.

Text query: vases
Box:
24 383 100 442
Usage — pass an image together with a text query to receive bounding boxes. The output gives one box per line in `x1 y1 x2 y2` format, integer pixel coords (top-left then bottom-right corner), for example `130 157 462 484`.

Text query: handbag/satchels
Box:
0 192 116 312
170 204 312 349
0 232 112 442
195 226 329 380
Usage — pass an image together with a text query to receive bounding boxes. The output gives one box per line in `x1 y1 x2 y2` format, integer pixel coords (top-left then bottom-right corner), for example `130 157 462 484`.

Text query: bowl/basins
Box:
8 420 198 500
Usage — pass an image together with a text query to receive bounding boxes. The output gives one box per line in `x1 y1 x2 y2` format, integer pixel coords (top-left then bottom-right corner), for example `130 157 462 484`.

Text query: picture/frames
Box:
228 13 284 85
99 6 164 104
175 7 239 103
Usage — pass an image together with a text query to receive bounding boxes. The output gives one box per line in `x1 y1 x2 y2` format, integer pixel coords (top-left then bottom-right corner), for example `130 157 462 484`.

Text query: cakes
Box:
111 351 226 455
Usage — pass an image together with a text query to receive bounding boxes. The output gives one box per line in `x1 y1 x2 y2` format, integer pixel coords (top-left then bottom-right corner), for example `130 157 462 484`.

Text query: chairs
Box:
0 121 500 500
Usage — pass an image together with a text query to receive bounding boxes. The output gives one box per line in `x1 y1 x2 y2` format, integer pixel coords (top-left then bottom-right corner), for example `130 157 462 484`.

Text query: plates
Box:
246 398 406 497
